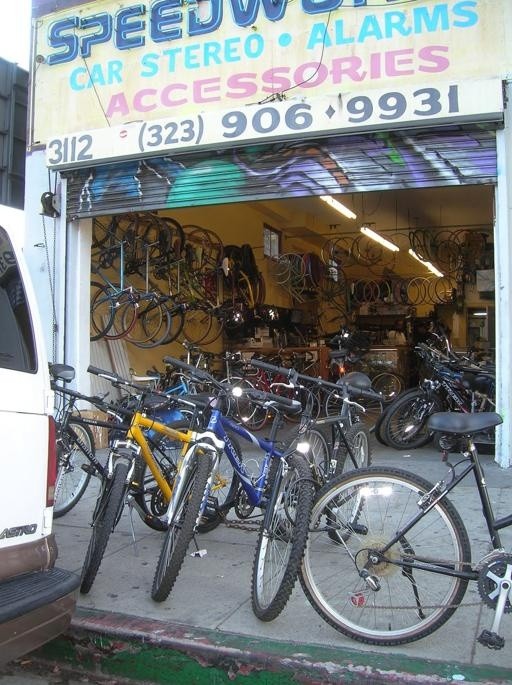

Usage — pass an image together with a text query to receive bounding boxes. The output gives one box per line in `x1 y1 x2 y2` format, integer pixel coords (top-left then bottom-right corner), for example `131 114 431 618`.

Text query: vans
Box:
0 227 82 670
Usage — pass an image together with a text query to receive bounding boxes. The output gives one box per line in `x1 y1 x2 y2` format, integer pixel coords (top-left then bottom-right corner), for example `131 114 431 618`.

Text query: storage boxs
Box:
68 409 109 450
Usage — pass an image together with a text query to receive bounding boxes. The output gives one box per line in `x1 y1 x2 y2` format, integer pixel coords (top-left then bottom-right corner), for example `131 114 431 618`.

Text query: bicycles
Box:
90 214 263 348
